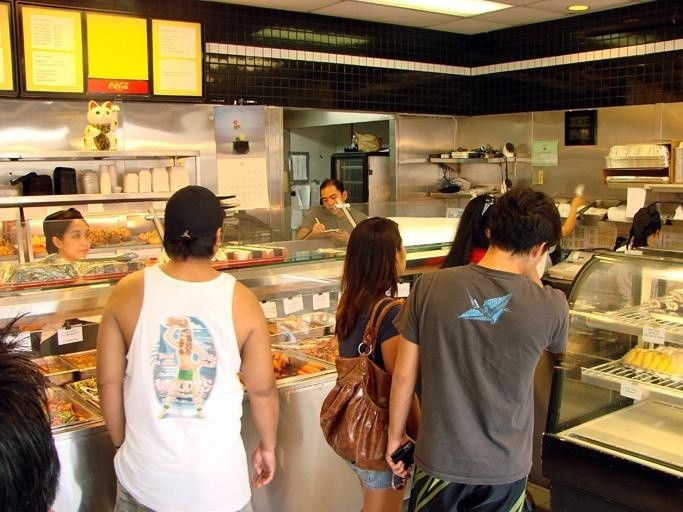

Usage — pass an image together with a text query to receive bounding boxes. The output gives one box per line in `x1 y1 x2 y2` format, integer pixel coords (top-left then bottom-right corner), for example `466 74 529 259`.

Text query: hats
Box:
165 185 226 239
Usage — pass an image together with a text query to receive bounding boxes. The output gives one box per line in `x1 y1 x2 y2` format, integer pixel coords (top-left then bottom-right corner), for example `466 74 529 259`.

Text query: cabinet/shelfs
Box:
543 254 683 511
429 156 513 200
0 149 198 204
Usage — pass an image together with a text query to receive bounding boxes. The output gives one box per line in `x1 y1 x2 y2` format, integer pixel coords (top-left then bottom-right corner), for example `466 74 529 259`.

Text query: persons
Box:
294 177 367 247
381 184 571 511
156 324 209 421
94 182 283 512
605 206 661 299
439 191 494 269
43 206 92 259
0 308 64 512
333 214 422 512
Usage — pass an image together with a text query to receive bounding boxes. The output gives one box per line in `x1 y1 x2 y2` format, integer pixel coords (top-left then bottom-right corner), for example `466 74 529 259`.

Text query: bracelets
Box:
114 446 119 449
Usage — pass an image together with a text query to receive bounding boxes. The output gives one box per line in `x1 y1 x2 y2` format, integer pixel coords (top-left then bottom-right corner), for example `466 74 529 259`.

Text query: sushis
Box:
648 291 683 317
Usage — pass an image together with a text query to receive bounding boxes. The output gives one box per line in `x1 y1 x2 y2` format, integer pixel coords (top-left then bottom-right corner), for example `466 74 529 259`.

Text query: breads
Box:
624 347 683 376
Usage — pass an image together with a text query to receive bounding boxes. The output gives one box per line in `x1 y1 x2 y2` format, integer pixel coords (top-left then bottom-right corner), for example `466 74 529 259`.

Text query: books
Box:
306 228 344 240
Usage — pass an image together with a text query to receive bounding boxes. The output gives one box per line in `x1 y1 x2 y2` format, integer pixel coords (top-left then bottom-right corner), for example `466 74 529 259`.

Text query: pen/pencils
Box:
315 217 320 224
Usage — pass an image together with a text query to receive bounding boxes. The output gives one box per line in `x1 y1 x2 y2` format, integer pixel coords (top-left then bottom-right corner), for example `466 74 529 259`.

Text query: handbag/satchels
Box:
321 354 421 472
